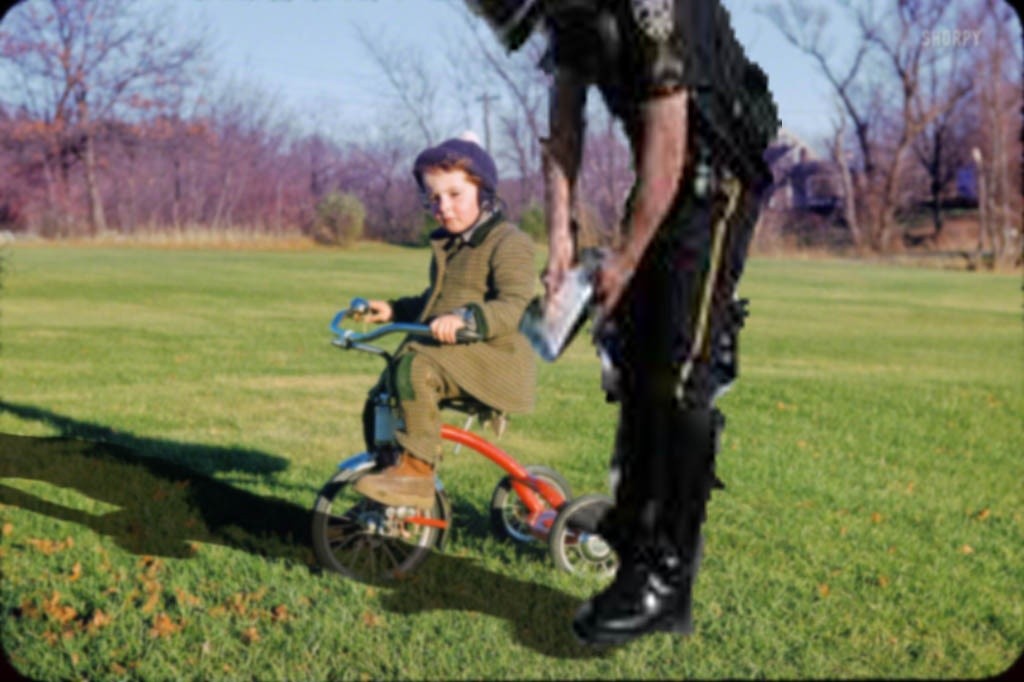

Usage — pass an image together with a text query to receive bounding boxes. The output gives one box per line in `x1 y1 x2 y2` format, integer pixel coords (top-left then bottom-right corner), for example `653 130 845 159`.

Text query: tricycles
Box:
309 299 625 594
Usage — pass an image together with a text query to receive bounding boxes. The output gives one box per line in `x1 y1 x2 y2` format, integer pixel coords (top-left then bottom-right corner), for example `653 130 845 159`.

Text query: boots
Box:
572 407 730 646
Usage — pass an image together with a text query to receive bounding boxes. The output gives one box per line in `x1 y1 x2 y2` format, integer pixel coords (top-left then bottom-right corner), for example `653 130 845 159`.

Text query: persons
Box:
324 138 540 557
466 0 784 652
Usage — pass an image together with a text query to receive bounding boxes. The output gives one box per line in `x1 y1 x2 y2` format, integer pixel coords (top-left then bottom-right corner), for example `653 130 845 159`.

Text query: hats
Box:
412 138 500 201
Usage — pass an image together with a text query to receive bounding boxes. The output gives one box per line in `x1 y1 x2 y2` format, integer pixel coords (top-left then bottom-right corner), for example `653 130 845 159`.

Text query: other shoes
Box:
355 468 436 510
